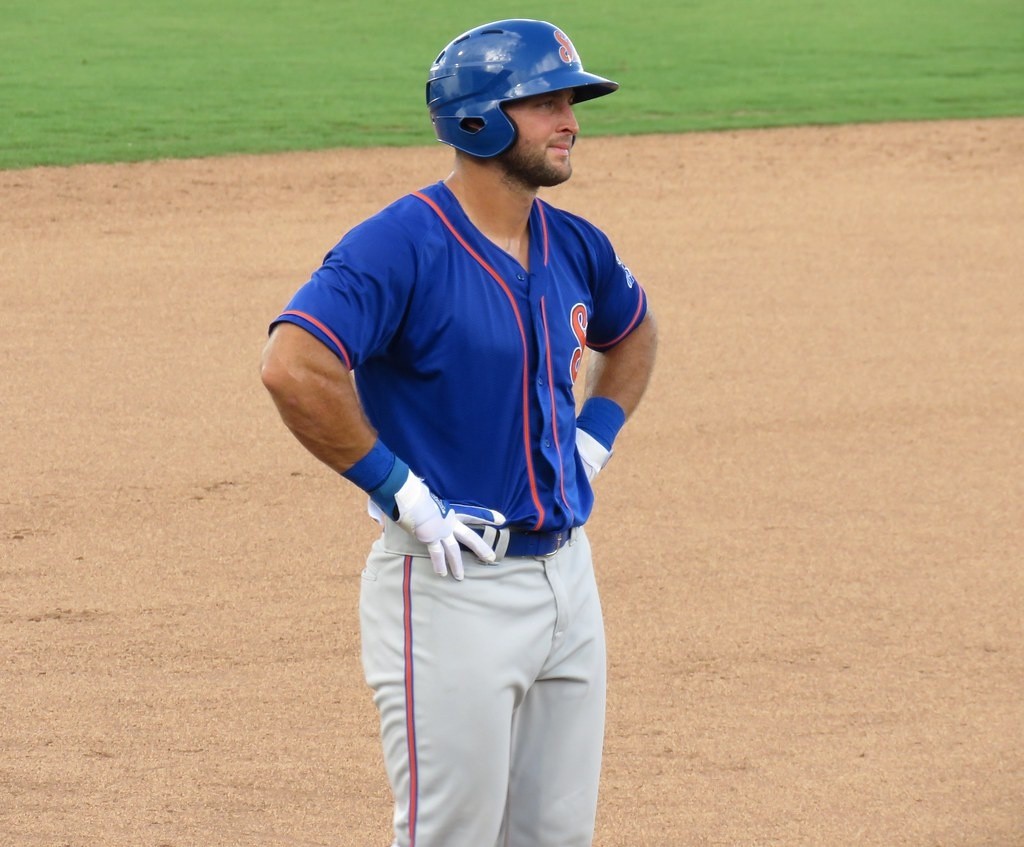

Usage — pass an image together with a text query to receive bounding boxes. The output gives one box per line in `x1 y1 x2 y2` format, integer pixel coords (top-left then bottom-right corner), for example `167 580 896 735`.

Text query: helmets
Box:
425 19 619 159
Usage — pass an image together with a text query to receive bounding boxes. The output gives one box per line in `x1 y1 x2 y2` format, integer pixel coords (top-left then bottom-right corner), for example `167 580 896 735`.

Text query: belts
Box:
457 528 570 557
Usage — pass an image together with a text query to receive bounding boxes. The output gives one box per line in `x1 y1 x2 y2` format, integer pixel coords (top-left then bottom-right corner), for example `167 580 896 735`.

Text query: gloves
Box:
575 426 613 484
367 468 506 582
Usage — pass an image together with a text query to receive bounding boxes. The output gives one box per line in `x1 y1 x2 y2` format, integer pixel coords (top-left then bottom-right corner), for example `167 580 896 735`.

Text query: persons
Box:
257 18 660 847
